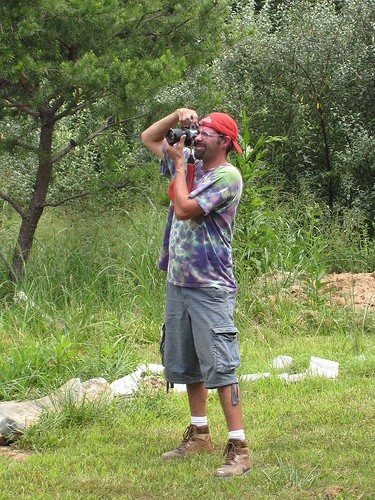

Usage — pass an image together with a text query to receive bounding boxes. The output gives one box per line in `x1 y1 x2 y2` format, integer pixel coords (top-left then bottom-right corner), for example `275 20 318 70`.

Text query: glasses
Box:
195 129 224 139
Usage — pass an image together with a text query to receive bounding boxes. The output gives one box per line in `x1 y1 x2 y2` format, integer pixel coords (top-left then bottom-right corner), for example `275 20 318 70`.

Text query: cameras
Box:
166 119 198 146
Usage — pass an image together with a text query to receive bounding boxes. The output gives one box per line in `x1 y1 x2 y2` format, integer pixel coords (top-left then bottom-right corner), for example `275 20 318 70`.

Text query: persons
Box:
141 108 251 478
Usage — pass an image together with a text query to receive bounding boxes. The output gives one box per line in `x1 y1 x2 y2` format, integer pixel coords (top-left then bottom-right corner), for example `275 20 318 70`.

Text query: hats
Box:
198 112 243 154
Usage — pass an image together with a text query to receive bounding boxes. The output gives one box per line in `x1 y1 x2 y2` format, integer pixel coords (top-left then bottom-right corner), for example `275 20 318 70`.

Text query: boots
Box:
213 438 251 477
162 424 213 459
